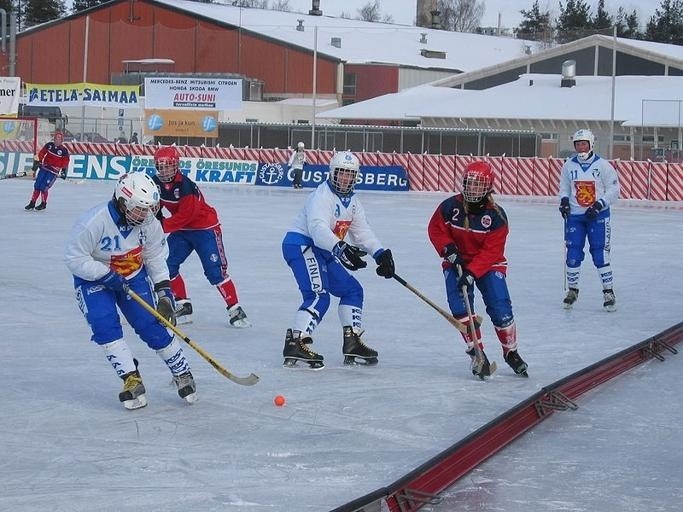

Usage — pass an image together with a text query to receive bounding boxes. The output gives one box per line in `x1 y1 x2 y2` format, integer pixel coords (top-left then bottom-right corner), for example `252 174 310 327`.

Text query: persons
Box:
559 129 619 306
287 142 305 189
428 162 529 376
151 146 247 324
282 150 378 361
64 171 196 402
129 133 139 145
25 131 69 210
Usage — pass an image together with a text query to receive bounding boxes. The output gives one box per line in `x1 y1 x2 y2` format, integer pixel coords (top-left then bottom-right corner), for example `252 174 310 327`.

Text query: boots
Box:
284 328 324 361
343 326 378 358
294 184 302 188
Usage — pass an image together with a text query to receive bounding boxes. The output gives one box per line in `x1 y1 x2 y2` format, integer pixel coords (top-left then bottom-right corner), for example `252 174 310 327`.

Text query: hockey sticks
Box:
41 166 86 185
455 263 496 380
122 283 260 385
379 263 483 334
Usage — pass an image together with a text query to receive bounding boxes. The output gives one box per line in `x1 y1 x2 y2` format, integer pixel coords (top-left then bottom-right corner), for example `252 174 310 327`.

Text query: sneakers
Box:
226 304 246 324
603 289 615 307
175 298 192 317
563 288 579 304
504 344 528 373
119 358 145 402
25 201 47 210
174 372 196 398
465 346 489 376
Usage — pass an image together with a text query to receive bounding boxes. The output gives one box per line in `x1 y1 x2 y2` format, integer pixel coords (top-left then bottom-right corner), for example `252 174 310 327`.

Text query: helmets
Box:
298 142 304 147
54 133 63 140
154 147 180 166
573 129 594 150
115 173 160 212
330 151 359 171
461 163 494 186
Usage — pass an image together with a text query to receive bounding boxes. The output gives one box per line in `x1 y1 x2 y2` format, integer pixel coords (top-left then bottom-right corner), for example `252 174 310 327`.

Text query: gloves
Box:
373 249 395 278
585 199 605 218
443 243 464 266
332 241 367 270
456 272 474 293
60 168 66 179
38 160 42 168
559 197 571 218
100 270 133 301
156 287 177 326
